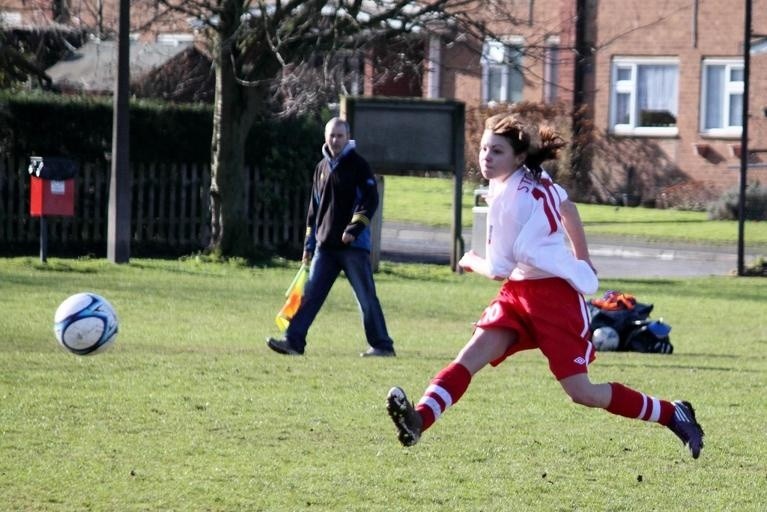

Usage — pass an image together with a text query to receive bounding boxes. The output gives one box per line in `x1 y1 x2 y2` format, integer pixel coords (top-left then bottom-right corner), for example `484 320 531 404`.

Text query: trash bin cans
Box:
471 189 488 258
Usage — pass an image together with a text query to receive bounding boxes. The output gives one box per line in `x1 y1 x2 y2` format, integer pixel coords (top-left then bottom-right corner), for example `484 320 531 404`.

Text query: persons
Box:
266 117 396 356
387 111 704 459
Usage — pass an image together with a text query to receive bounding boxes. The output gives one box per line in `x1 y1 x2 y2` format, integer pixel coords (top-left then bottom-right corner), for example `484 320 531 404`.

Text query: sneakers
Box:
360 347 396 358
384 386 422 447
667 398 704 460
266 337 304 356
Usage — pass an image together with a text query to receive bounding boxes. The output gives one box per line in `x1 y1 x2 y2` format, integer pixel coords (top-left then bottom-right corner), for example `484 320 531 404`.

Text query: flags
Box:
275 264 306 333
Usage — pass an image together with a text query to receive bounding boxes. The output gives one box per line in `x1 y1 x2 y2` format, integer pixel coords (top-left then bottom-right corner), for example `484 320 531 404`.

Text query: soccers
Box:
592 326 619 351
55 294 118 356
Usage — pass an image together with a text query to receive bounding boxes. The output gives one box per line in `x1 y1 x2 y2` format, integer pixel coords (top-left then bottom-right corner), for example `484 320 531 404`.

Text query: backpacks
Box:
622 319 673 355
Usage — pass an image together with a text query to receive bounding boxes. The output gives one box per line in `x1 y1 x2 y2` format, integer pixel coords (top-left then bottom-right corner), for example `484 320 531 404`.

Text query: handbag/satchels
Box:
582 298 654 351
